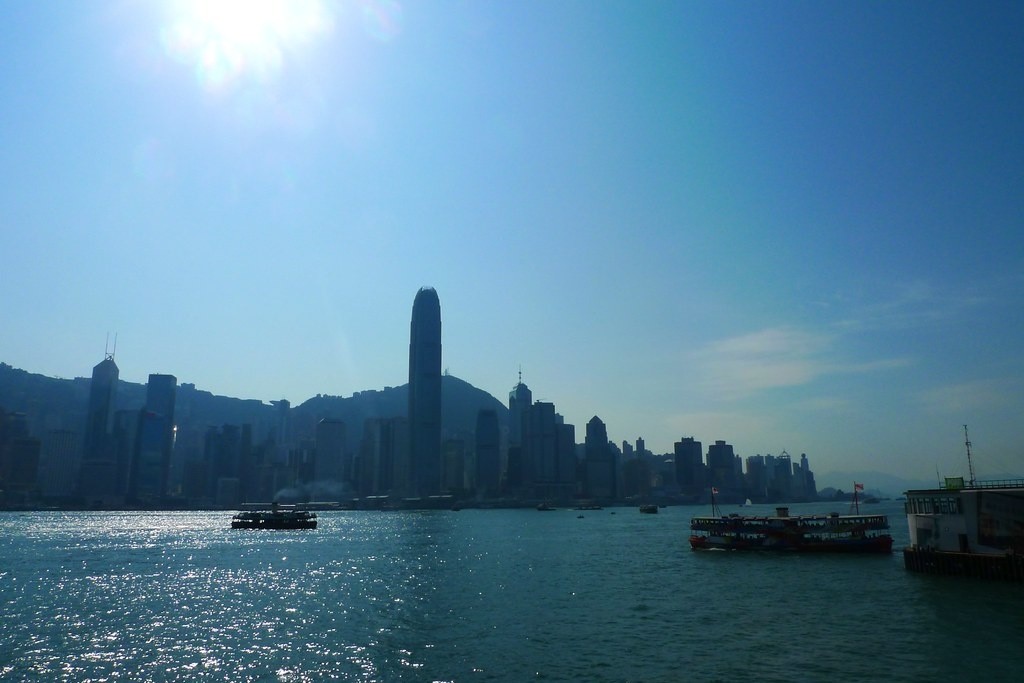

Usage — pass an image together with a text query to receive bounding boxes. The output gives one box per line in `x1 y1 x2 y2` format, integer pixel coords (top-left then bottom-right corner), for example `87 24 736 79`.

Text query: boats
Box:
231 500 318 529
573 503 604 510
577 513 584 518
658 504 666 508
639 504 657 513
536 493 557 511
687 481 895 554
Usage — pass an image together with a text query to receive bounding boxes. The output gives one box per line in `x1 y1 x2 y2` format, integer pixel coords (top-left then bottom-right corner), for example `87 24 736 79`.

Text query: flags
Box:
855 484 863 490
